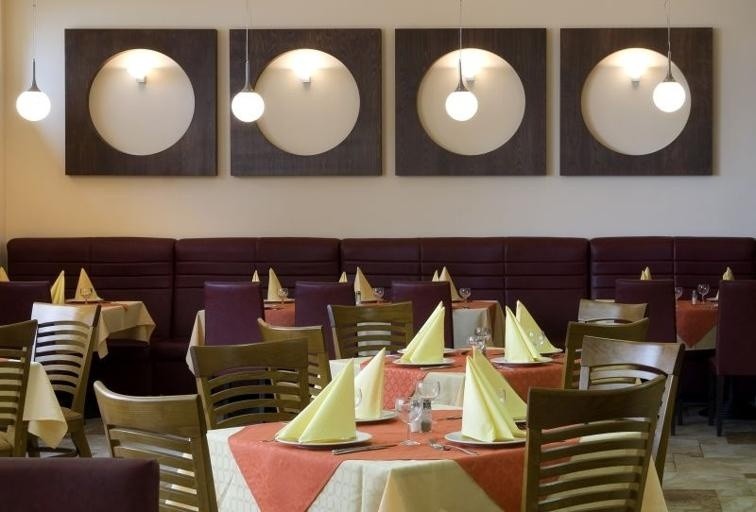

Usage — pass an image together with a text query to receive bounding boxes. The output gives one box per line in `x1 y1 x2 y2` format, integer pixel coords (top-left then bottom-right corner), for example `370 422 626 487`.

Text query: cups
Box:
355 386 363 407
413 397 434 434
495 388 506 401
529 330 545 353
470 326 493 352
675 287 683 300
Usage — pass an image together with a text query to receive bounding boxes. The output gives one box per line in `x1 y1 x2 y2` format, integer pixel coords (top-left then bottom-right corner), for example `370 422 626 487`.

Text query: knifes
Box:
332 444 398 454
420 364 462 371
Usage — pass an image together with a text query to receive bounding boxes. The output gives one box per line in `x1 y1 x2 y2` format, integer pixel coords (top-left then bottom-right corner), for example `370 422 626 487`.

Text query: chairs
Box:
0 236 756 511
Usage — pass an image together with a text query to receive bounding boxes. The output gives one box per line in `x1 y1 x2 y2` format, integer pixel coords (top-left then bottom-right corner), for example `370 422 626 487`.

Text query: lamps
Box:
445 1 478 122
653 1 687 113
12 1 53 124
229 1 266 126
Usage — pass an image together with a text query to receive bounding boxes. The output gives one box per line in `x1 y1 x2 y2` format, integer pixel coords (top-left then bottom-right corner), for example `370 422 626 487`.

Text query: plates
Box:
354 410 400 423
540 348 563 355
67 299 103 303
264 299 295 304
511 418 527 423
361 299 383 303
490 355 554 366
444 431 524 446
391 358 457 365
274 431 374 447
396 347 471 355
707 297 719 301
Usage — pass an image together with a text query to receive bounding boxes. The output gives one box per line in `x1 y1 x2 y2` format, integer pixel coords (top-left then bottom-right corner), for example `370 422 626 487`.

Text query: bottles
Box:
692 290 697 305
356 290 362 305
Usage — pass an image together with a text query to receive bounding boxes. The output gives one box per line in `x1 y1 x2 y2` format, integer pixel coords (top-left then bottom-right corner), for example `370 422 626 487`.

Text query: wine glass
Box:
395 397 422 446
372 288 384 303
697 283 709 304
416 381 441 426
277 287 289 306
460 288 471 307
80 286 93 306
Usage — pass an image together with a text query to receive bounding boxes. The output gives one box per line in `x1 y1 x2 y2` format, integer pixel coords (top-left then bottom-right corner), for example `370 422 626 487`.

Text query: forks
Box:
427 437 479 456
